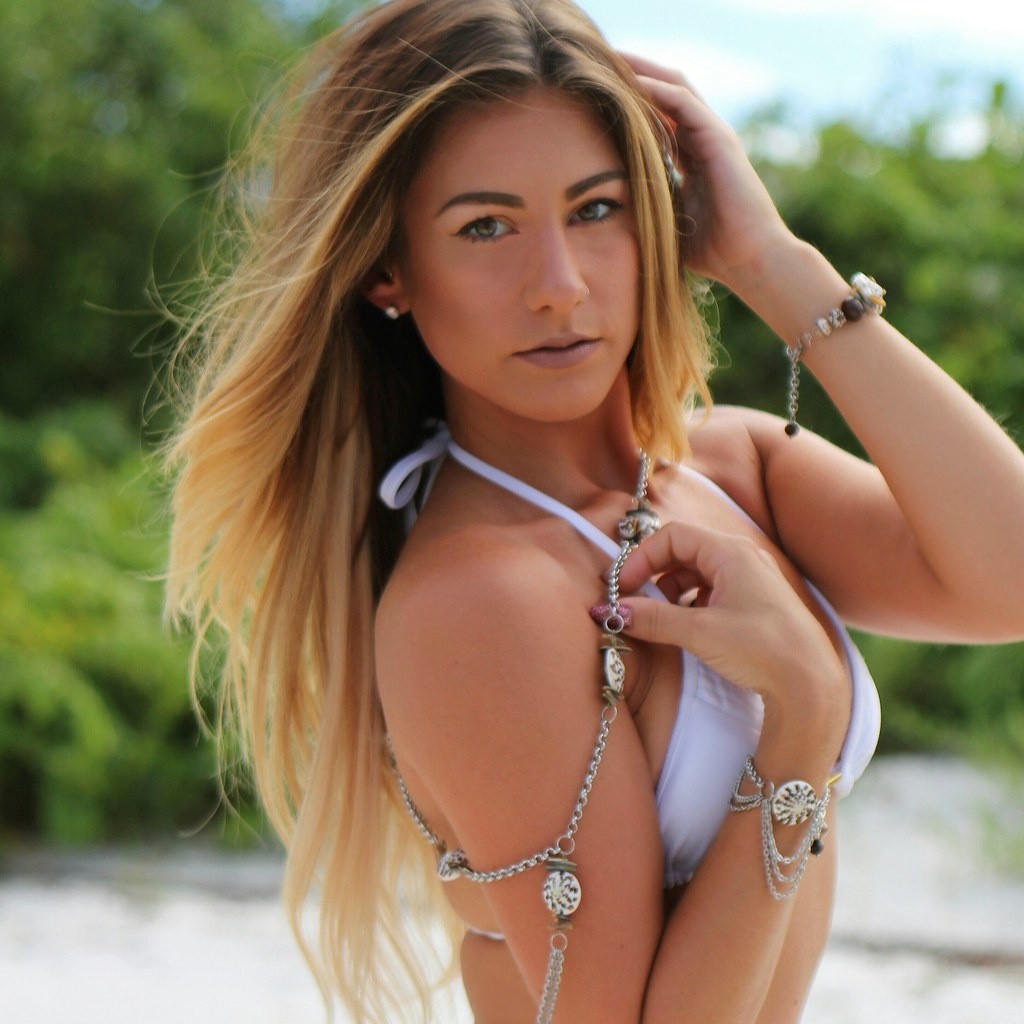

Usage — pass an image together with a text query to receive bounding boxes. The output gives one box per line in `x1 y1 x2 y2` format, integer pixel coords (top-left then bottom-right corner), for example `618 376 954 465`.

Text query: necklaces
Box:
383 444 661 1024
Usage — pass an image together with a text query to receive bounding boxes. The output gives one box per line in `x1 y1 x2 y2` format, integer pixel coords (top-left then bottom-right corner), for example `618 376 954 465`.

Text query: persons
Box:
131 1 1024 1024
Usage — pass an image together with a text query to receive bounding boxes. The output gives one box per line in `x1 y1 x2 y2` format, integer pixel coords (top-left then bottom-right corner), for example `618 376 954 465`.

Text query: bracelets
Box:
727 754 842 900
784 272 886 438
